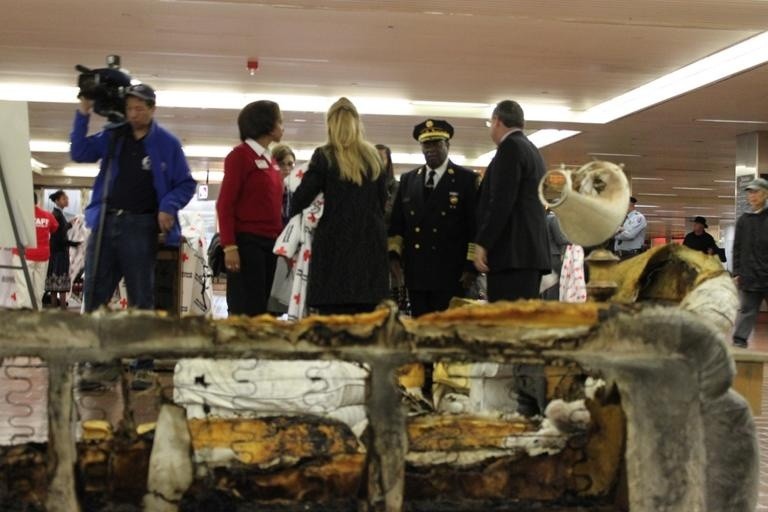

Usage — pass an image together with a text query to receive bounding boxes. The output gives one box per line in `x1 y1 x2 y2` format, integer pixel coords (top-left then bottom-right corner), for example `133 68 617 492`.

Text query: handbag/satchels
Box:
207 233 228 278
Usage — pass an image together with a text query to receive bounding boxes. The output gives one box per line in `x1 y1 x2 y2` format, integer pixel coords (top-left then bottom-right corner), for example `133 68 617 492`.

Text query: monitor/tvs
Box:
197 183 220 201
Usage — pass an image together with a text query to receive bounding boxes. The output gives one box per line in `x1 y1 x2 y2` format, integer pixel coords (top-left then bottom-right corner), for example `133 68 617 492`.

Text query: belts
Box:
615 249 641 257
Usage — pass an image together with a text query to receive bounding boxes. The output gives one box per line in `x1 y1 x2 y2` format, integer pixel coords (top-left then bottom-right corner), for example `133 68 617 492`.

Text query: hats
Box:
690 216 708 228
126 84 156 102
744 178 767 192
413 119 454 144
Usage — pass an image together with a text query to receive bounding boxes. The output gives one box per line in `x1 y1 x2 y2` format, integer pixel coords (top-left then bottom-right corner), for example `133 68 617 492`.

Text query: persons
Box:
733 178 768 348
12 191 59 312
683 217 719 256
44 192 83 309
70 84 197 312
216 97 647 320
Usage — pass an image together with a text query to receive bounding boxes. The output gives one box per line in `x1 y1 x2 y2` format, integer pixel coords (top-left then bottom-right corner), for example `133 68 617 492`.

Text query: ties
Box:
424 171 435 199
